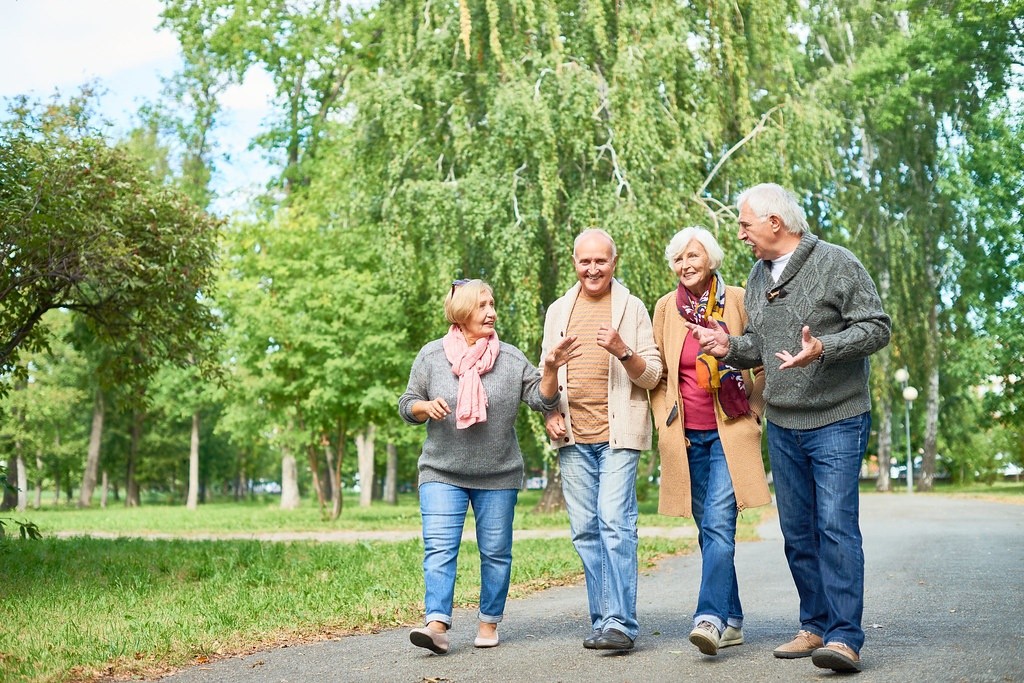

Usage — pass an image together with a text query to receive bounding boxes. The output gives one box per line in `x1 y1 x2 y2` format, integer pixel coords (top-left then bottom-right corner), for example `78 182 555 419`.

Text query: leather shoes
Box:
595 628 634 649
583 629 602 649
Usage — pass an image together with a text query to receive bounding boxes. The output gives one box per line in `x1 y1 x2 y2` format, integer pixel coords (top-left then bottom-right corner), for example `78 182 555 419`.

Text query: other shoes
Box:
812 641 861 674
688 621 721 656
409 627 450 654
718 625 744 647
473 625 499 648
772 629 824 660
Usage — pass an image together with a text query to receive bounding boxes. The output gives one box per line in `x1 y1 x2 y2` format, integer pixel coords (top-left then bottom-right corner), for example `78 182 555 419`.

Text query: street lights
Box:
895 368 919 493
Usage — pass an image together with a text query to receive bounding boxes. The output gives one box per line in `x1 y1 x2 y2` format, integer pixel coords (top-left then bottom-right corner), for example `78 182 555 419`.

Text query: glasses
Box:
451 278 470 298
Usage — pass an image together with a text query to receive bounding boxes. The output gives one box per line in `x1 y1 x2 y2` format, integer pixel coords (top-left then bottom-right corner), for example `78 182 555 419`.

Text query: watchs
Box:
617 347 634 361
817 350 825 365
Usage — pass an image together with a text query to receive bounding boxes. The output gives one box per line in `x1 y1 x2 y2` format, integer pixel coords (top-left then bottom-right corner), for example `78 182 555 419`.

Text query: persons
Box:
536 228 662 649
397 278 584 656
685 183 893 673
648 227 773 656
